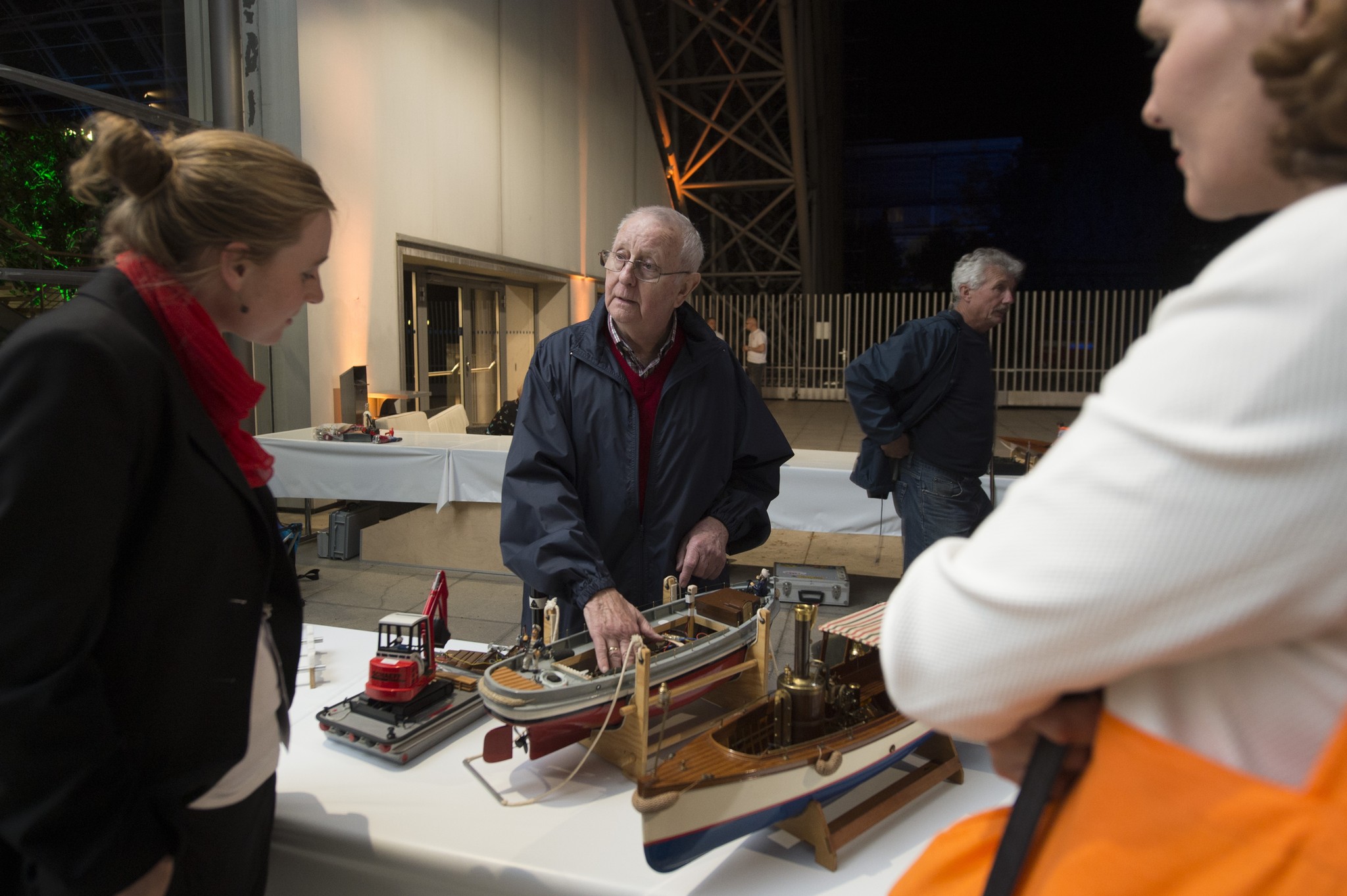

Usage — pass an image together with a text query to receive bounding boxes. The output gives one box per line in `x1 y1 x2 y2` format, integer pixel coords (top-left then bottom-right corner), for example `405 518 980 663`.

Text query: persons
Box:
872 0 1347 894
842 246 1025 577
705 316 725 342
499 202 801 679
0 103 342 896
742 317 768 401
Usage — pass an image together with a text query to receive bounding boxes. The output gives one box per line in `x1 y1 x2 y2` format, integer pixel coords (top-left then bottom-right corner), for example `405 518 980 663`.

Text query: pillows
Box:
428 404 470 434
375 411 430 434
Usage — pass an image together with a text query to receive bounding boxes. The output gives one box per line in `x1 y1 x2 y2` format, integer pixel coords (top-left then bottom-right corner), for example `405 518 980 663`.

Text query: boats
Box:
632 593 938 875
478 568 781 762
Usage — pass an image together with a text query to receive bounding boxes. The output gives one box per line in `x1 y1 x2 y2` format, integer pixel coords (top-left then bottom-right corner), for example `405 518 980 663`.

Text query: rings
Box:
608 646 620 654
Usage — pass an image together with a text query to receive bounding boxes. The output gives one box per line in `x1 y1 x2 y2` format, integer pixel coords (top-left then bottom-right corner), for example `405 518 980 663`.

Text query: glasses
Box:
598 250 690 283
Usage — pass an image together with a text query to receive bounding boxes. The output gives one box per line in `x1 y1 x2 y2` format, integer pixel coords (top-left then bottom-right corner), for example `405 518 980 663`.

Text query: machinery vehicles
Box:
316 569 491 765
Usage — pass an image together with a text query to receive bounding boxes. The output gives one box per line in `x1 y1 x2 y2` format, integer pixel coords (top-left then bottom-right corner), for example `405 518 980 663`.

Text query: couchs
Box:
372 406 493 436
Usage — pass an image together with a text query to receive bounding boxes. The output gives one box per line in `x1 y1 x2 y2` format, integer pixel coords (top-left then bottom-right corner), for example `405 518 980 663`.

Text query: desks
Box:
258 421 1032 548
262 618 1024 896
